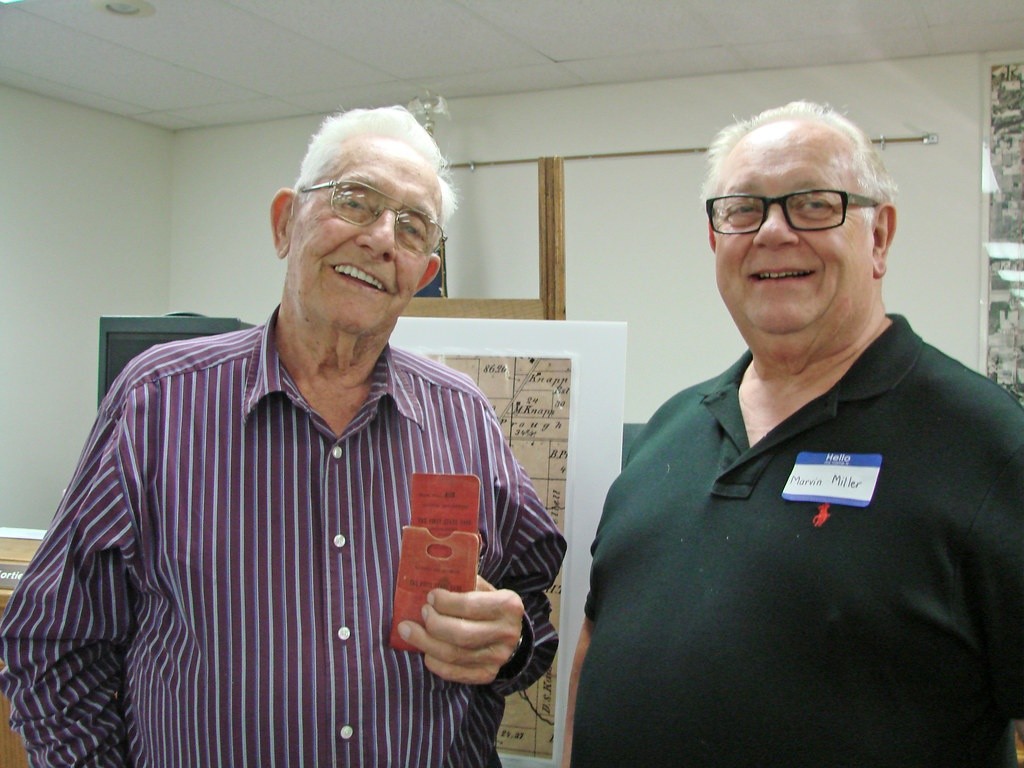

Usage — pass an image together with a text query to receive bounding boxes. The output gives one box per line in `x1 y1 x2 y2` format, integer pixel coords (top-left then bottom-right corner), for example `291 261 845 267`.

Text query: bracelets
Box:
509 634 523 662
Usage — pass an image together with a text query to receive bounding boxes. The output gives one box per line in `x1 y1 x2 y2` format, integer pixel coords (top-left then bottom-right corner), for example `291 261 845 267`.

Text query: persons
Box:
561 98 1024 768
1 105 566 768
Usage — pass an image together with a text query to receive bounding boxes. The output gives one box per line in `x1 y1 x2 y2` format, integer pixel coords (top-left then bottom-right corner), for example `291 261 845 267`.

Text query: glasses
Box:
706 188 884 234
296 177 448 256
